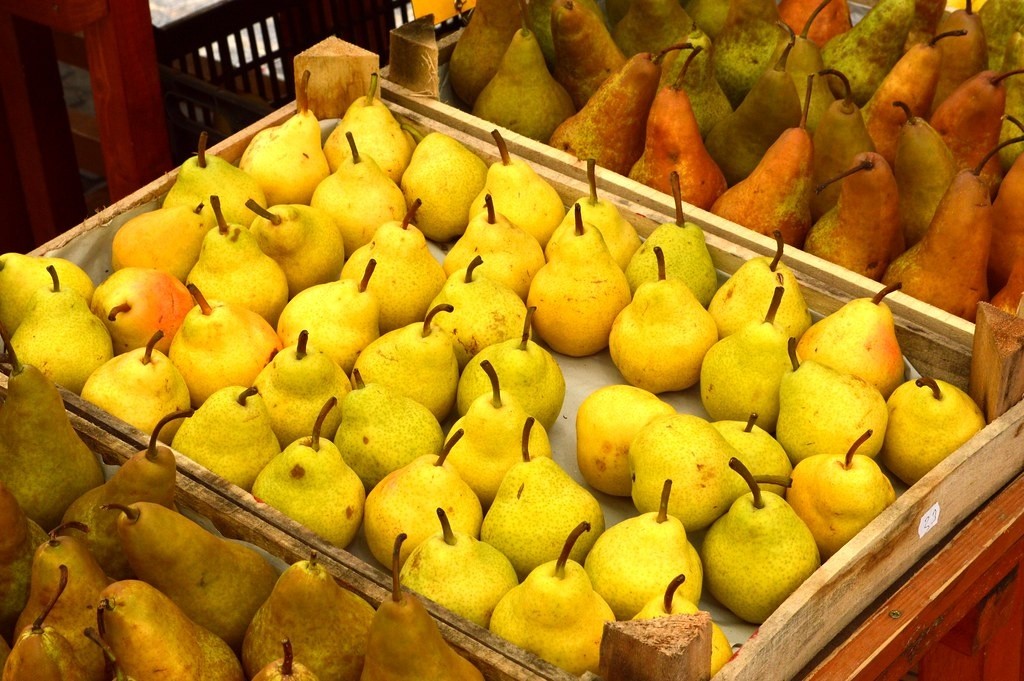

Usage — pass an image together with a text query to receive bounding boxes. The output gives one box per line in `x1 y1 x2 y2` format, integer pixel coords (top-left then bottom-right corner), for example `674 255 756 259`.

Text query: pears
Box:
0 0 1024 681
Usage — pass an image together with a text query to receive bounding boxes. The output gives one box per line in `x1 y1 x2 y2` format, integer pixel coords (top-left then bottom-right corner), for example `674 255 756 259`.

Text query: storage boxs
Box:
0 0 1024 678
